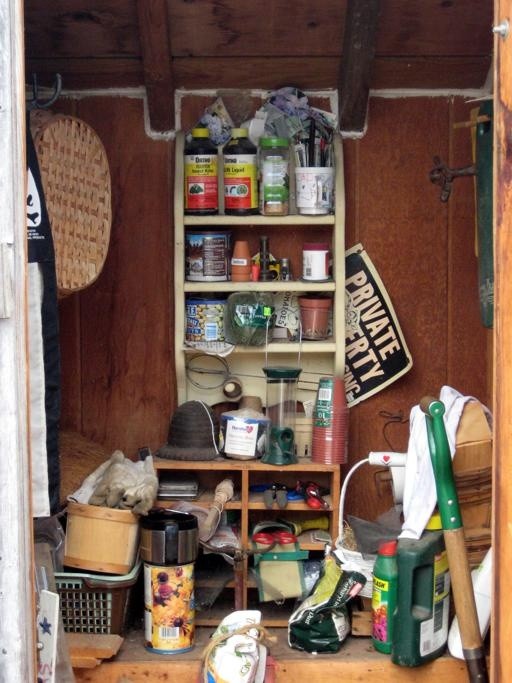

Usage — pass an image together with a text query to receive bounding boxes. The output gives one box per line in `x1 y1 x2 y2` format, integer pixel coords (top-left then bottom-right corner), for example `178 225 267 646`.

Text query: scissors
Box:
252 529 298 545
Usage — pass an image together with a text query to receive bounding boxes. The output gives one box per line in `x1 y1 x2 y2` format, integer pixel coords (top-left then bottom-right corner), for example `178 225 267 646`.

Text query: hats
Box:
157 400 223 462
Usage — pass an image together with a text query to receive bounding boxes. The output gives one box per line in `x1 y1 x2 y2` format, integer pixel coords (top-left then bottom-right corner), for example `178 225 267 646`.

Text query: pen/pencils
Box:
295 119 325 166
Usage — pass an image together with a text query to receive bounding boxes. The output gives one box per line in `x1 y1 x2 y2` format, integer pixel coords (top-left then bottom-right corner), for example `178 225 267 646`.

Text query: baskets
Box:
51 554 145 639
28 107 119 302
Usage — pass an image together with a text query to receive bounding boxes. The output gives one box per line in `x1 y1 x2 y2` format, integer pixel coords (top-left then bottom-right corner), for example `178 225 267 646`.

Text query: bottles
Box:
184 127 219 214
261 365 301 465
372 540 396 656
300 241 332 284
257 136 291 216
223 126 259 215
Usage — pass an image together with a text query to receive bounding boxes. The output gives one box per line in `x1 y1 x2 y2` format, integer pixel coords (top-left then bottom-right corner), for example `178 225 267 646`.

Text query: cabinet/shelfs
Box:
171 126 342 457
145 455 345 627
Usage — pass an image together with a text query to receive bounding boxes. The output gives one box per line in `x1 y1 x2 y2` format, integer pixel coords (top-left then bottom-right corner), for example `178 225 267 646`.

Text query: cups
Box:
311 376 350 465
296 162 335 214
232 240 250 275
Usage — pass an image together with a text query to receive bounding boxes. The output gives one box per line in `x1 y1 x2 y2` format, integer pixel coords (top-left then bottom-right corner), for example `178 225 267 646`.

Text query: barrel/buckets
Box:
142 563 195 654
391 503 453 667
63 502 141 575
